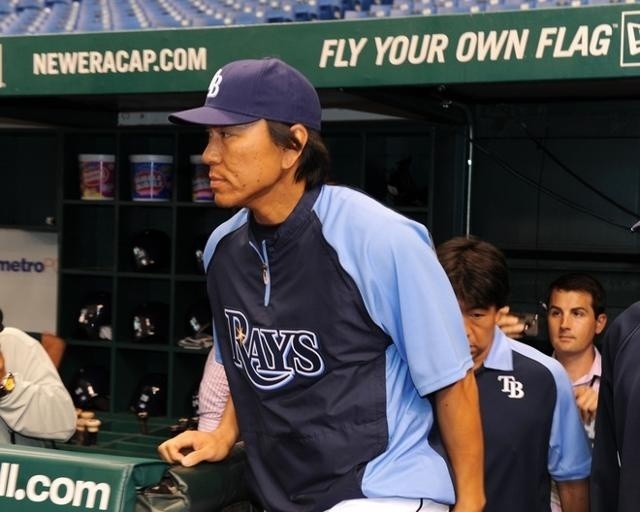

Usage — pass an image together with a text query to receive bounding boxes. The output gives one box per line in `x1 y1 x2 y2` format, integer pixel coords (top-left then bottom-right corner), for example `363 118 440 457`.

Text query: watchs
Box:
0 369 16 398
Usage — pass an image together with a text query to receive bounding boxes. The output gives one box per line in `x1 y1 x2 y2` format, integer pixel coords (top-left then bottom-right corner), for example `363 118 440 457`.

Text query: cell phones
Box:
506 311 539 337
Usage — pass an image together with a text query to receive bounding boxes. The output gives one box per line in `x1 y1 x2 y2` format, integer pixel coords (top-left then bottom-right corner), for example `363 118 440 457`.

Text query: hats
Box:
168 57 322 132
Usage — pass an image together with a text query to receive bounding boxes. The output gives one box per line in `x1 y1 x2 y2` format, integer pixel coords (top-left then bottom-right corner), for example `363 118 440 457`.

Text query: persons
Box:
496 271 608 512
427 237 593 512
157 56 488 512
0 308 79 446
587 220 640 512
196 344 231 433
25 330 66 370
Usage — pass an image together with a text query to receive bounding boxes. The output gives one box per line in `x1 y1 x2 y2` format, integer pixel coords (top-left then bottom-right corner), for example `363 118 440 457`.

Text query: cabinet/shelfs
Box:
50 119 438 424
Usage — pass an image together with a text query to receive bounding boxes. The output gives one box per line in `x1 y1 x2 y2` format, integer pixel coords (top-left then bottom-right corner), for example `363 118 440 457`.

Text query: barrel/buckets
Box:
129 155 172 202
190 155 215 203
79 154 116 201
